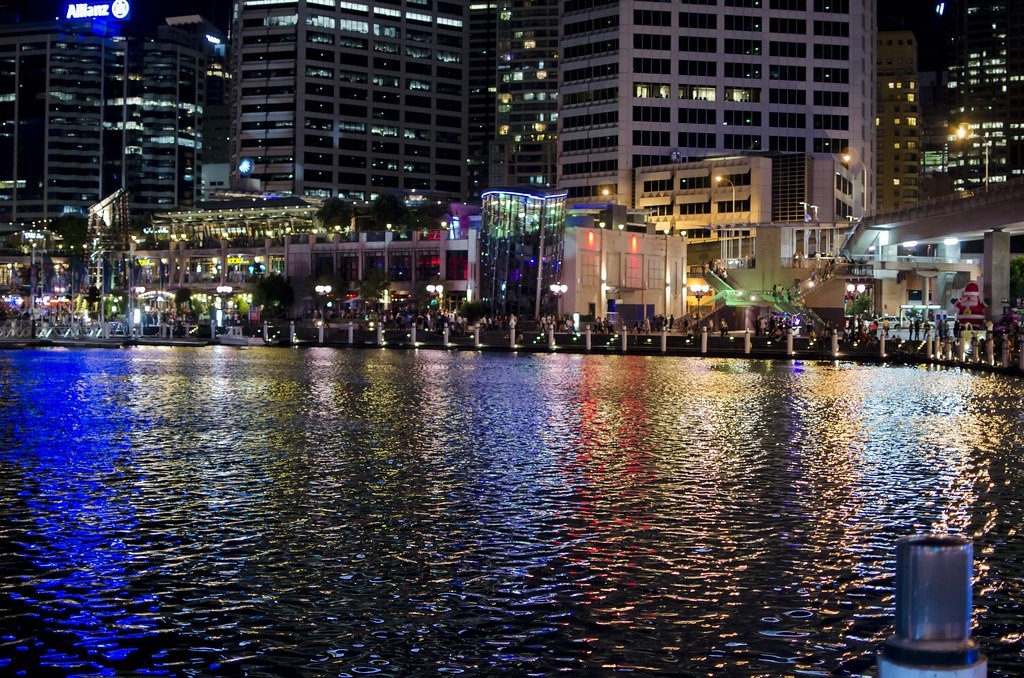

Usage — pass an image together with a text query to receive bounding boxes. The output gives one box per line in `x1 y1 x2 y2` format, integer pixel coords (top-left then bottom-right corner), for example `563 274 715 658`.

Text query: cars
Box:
929 316 955 330
875 315 911 330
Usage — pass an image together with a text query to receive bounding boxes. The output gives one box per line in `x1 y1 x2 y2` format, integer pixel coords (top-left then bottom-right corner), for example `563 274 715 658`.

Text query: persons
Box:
773 279 801 304
142 308 195 334
683 318 688 334
536 313 570 332
721 318 729 337
634 315 674 333
751 251 755 268
593 316 609 334
395 309 467 332
810 255 839 284
480 314 510 331
709 258 728 282
909 319 949 341
808 314 889 344
839 254 864 275
993 322 1023 359
754 316 789 337
950 282 989 331
307 308 346 319
953 318 993 344
708 317 713 334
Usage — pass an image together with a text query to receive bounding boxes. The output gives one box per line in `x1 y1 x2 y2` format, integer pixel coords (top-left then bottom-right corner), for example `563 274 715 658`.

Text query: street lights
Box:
216 285 233 328
846 284 865 334
548 284 568 332
314 285 332 322
956 128 989 192
425 284 443 331
689 283 709 329
714 176 735 237
843 155 866 215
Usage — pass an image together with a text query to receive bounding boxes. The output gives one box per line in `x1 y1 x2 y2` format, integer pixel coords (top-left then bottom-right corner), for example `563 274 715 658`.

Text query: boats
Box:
216 325 265 346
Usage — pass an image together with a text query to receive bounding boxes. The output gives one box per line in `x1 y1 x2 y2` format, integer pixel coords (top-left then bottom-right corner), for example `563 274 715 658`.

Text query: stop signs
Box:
963 282 979 299
